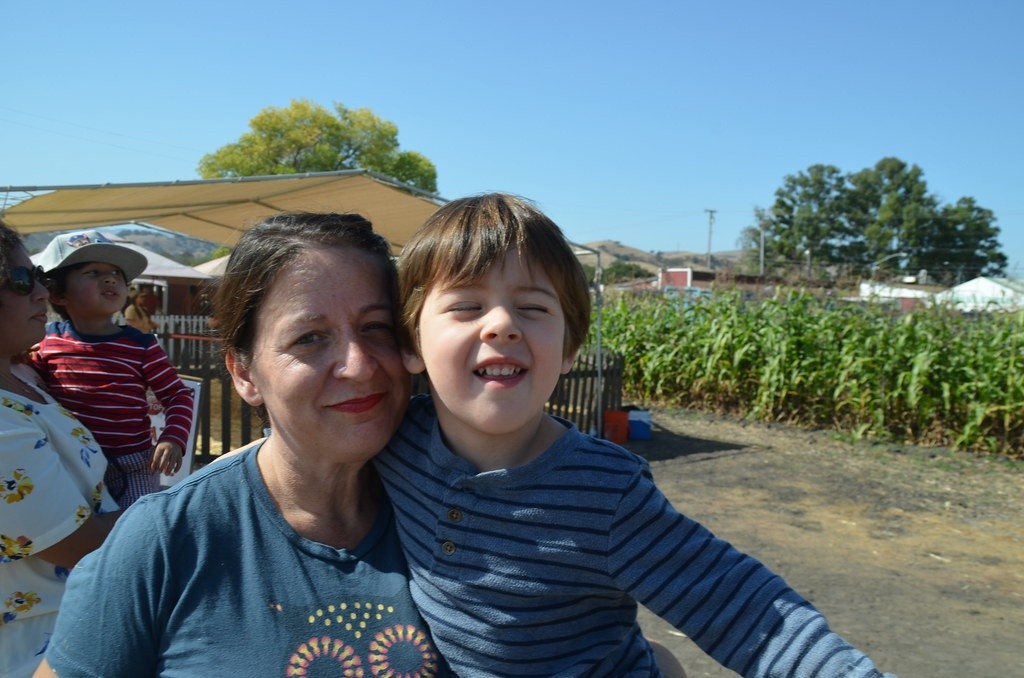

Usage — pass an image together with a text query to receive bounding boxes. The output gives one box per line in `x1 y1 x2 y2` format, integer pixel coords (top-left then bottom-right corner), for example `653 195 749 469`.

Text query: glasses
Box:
0 265 45 296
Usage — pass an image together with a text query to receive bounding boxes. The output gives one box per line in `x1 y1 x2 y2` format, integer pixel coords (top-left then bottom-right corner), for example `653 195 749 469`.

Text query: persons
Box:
30 193 896 678
142 284 161 315
125 289 160 334
0 216 193 678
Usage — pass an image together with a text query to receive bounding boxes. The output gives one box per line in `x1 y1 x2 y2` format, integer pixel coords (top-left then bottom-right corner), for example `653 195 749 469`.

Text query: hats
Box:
130 290 147 297
39 229 148 282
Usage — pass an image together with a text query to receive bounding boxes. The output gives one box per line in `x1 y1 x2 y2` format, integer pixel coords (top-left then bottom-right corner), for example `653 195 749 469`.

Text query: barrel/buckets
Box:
603 411 627 443
627 409 651 440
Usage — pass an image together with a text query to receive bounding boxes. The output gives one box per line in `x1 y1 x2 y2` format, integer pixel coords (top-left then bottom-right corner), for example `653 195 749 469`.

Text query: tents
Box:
193 254 232 277
28 232 214 279
0 168 602 436
925 276 1024 313
